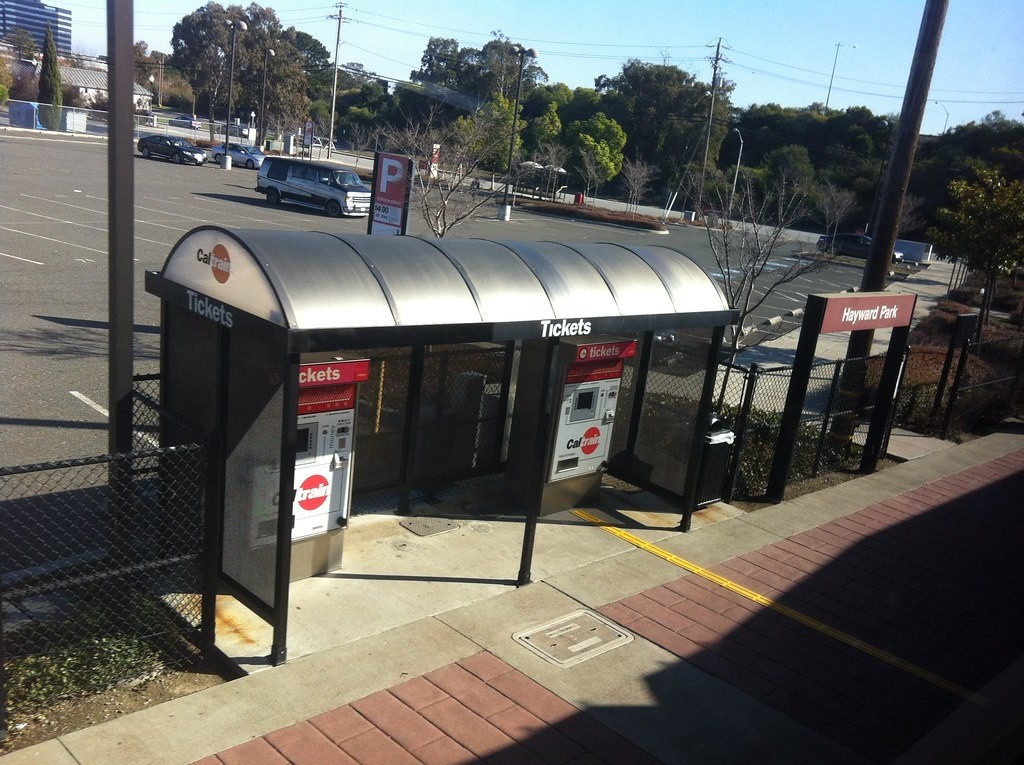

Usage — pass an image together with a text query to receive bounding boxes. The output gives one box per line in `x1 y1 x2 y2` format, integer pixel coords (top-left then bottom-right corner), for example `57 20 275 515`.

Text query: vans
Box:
254 155 373 217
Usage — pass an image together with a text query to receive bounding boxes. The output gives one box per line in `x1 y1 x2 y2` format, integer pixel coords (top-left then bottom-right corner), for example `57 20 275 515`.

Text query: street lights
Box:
933 100 950 135
823 42 858 113
147 73 156 126
498 40 538 223
256 46 276 146
724 126 745 223
694 90 716 219
223 18 248 154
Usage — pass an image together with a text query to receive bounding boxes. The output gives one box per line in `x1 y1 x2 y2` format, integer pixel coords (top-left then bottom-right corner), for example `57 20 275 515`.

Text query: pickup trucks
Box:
170 115 202 130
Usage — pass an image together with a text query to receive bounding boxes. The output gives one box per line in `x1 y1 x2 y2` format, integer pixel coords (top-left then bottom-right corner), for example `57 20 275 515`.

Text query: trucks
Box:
894 239 933 270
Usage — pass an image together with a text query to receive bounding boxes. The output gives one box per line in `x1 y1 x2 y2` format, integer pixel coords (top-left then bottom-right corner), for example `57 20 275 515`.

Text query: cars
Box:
217 124 243 137
211 141 266 169
258 127 335 151
816 231 903 263
137 134 209 166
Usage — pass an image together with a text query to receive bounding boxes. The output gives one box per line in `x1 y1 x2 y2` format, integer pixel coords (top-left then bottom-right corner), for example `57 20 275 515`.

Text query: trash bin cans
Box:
496 201 512 221
662 414 739 510
573 191 584 206
683 210 696 222
